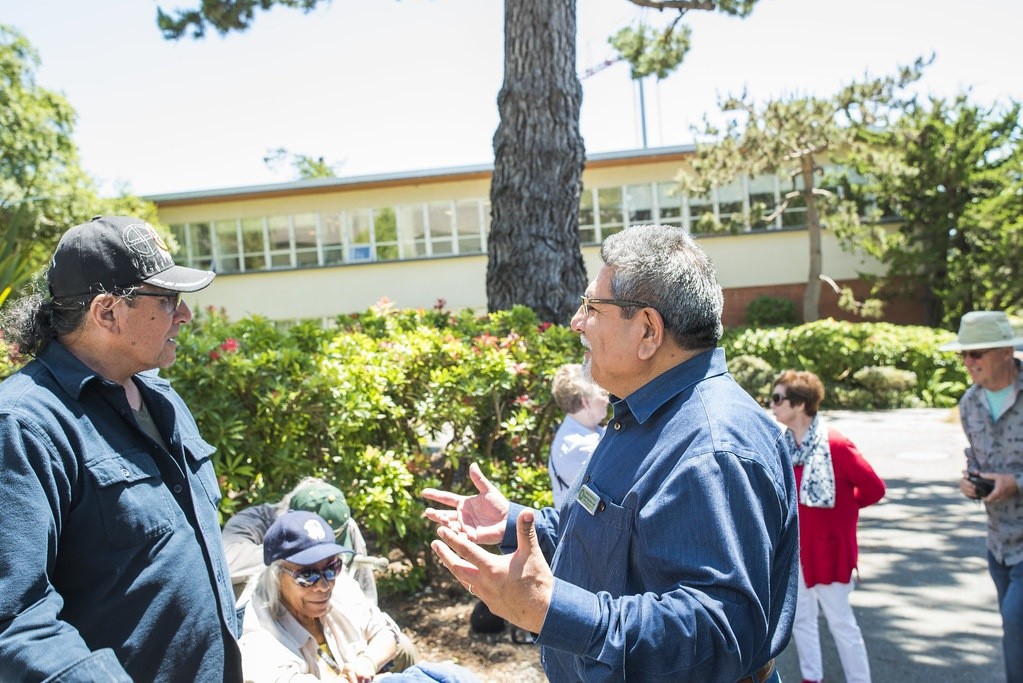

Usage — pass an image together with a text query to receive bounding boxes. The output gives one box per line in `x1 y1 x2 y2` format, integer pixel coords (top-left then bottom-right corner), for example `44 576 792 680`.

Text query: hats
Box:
289 482 353 565
262 510 357 566
48 215 216 297
938 311 1023 351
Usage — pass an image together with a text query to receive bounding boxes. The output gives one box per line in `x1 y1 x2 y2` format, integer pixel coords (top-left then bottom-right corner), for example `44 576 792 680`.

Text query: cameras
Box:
970 477 994 498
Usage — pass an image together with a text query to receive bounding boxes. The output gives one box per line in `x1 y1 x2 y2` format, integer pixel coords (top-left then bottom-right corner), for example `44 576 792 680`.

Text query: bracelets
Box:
360 654 378 670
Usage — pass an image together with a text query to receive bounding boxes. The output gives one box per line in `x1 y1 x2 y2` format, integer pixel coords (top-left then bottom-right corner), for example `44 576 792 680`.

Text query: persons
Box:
548 363 610 510
223 476 379 608
0 214 242 683
421 224 799 683
769 370 886 683
238 508 481 683
937 311 1023 683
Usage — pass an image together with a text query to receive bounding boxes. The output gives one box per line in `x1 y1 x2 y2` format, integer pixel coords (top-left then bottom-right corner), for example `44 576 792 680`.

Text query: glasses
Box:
114 290 183 312
279 557 343 588
773 394 795 405
955 349 993 360
580 296 670 328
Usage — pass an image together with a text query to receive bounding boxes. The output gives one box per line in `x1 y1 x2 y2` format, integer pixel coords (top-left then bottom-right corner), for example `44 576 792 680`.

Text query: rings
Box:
468 584 474 595
